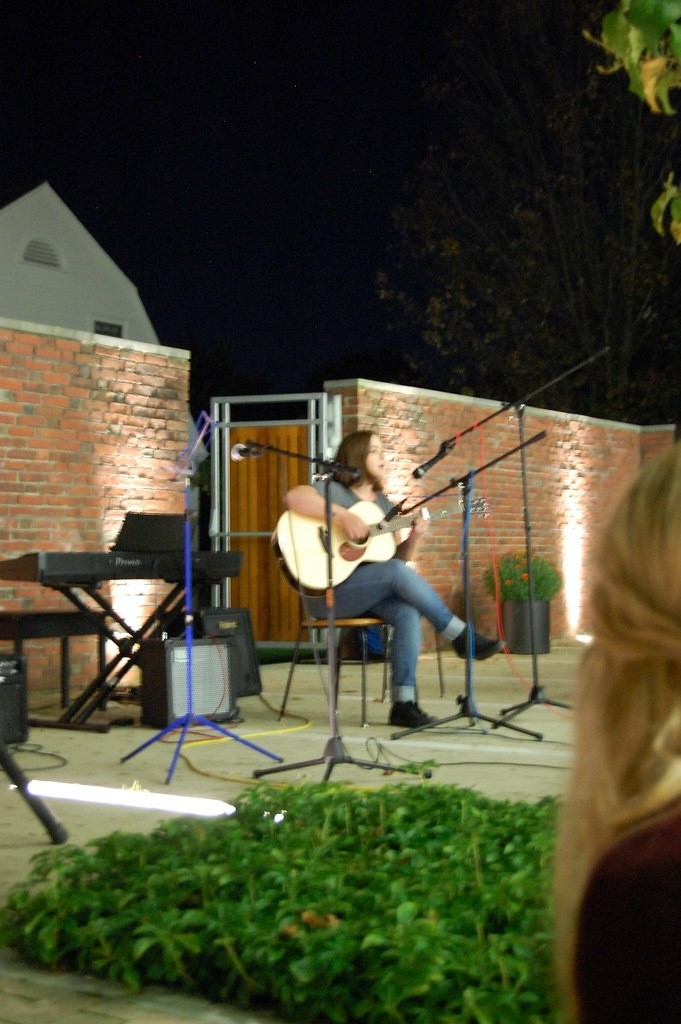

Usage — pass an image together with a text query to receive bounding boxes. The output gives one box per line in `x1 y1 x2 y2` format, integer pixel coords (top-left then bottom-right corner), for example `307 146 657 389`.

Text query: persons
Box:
284 432 508 728
557 448 681 1023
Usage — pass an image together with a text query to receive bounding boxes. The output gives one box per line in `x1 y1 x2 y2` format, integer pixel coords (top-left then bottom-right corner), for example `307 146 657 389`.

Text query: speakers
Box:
162 608 263 698
140 638 236 727
0 655 29 743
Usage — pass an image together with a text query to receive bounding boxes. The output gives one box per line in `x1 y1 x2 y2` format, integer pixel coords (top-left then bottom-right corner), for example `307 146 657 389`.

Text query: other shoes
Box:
454 629 506 661
390 701 436 729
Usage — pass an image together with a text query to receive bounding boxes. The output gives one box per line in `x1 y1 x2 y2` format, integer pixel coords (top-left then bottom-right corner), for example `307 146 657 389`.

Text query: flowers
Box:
482 548 562 600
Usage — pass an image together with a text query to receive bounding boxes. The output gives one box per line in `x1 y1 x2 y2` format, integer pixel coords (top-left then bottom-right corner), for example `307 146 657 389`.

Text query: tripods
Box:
388 348 611 742
248 440 432 789
120 410 283 786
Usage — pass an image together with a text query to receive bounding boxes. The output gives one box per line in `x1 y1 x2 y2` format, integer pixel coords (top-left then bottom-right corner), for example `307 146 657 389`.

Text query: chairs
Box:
278 596 447 726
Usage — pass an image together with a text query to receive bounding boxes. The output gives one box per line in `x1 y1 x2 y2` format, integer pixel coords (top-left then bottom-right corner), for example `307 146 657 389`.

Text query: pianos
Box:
0 550 243 582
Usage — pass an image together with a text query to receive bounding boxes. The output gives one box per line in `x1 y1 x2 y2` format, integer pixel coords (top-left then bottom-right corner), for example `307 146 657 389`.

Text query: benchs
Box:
1 610 109 712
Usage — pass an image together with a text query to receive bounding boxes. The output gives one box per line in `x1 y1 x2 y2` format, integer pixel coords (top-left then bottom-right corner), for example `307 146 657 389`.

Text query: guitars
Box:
271 495 491 599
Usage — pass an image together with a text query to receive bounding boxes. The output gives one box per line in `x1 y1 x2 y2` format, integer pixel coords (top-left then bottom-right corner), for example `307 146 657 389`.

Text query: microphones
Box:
230 443 263 462
377 498 407 530
412 443 456 480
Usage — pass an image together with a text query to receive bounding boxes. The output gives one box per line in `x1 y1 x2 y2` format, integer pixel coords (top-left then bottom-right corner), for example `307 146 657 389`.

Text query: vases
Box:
502 599 550 653
